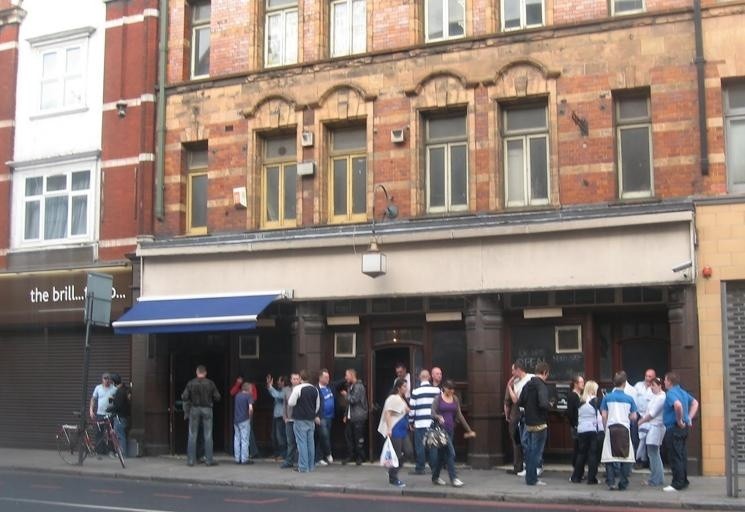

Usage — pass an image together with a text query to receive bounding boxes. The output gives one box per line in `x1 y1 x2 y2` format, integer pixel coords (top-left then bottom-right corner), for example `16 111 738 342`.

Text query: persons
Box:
504 362 555 486
377 363 475 487
108 374 130 457
567 369 698 491
90 373 117 455
232 375 259 464
181 365 222 466
267 368 368 472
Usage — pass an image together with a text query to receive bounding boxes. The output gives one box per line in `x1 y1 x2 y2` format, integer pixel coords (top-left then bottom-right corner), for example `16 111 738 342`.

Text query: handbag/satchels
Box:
422 421 449 449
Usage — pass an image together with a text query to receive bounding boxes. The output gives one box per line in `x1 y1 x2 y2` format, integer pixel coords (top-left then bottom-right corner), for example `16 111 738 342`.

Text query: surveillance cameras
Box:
683 268 689 279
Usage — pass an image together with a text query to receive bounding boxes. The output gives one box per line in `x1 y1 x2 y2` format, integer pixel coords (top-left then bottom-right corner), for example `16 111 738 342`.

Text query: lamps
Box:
357 182 398 279
115 96 129 119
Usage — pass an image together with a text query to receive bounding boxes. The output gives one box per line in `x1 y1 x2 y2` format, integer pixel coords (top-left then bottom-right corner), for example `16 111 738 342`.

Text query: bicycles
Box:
54 406 126 470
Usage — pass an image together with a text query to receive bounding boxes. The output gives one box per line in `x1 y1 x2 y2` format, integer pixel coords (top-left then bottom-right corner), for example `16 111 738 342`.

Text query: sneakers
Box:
450 479 464 487
431 477 446 486
389 480 405 489
186 449 362 473
505 468 690 492
